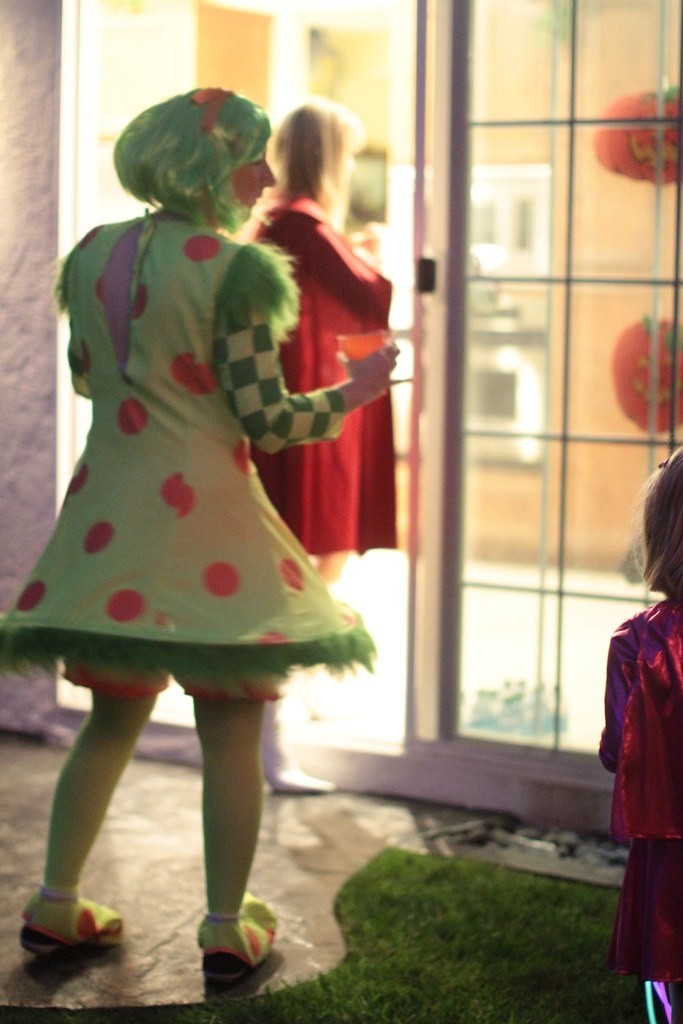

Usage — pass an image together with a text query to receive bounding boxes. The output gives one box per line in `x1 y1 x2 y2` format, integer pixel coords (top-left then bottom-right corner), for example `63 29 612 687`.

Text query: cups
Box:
338 328 392 401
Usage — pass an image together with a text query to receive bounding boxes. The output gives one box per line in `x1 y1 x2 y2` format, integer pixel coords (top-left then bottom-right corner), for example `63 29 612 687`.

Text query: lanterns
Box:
594 88 683 184
614 316 683 433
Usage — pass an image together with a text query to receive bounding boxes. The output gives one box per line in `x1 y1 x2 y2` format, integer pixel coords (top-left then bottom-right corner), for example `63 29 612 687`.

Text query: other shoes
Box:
268 767 336 796
200 893 277 983
20 895 123 955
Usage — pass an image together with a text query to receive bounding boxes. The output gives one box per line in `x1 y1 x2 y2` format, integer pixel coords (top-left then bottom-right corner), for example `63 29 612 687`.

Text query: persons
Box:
0 87 403 986
248 100 398 792
600 449 683 1024
